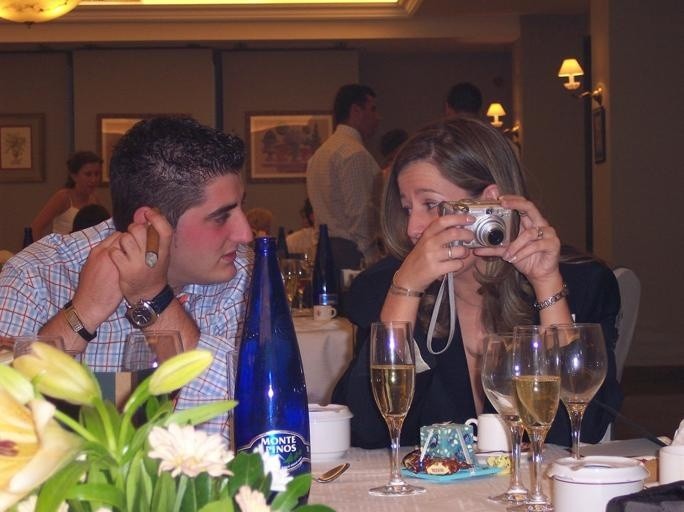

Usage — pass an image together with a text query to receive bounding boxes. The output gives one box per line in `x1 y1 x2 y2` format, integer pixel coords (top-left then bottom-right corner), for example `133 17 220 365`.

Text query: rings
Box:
537 226 543 239
448 247 452 258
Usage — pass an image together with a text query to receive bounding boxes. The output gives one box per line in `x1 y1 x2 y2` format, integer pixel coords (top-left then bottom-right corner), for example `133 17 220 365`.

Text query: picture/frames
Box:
245 110 336 184
96 113 193 188
0 114 46 183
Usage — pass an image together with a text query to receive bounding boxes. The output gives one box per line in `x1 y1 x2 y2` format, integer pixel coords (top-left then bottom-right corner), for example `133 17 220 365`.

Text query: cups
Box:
226 350 241 454
314 305 338 321
9 332 71 364
120 327 187 411
657 446 684 485
464 413 513 454
341 268 363 288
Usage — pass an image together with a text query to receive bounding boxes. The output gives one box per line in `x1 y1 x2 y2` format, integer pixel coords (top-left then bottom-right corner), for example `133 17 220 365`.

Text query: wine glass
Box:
295 260 311 314
547 322 611 458
366 319 429 499
279 260 298 315
512 324 562 511
479 333 539 508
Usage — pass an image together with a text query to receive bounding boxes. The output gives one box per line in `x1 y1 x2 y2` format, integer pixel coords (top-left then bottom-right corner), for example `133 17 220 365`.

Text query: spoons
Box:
309 460 350 489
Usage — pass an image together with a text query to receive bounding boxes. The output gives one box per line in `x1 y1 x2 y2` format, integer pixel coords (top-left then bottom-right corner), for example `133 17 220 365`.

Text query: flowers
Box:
0 341 335 512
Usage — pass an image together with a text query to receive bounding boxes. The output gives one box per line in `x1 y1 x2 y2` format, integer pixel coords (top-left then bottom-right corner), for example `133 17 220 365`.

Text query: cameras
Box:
442 199 511 250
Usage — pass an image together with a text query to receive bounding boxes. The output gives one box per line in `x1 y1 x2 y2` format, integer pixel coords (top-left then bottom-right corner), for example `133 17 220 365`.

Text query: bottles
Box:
20 226 34 249
233 238 311 512
275 227 288 258
309 224 342 319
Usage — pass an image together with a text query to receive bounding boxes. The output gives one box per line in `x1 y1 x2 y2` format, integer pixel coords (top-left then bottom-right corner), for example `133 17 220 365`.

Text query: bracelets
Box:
534 286 570 312
390 272 424 298
63 301 97 341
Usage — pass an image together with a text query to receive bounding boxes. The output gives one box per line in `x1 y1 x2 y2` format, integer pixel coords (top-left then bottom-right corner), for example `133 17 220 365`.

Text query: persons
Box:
30 150 103 242
72 204 110 232
332 113 622 450
306 83 385 289
0 113 255 450
446 82 483 118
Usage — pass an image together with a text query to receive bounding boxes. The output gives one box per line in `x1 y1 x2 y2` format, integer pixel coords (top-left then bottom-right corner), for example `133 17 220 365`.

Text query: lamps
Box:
558 59 584 91
486 103 506 127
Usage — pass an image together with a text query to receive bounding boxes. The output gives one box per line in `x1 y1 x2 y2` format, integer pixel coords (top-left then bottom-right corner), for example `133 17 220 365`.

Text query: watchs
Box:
125 284 173 329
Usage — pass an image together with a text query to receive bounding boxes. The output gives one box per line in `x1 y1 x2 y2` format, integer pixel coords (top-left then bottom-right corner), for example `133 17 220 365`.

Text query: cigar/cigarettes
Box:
145 207 162 267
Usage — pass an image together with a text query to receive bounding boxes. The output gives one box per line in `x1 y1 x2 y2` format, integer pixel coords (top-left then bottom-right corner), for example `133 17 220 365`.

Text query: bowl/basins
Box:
303 403 356 462
544 455 652 512
